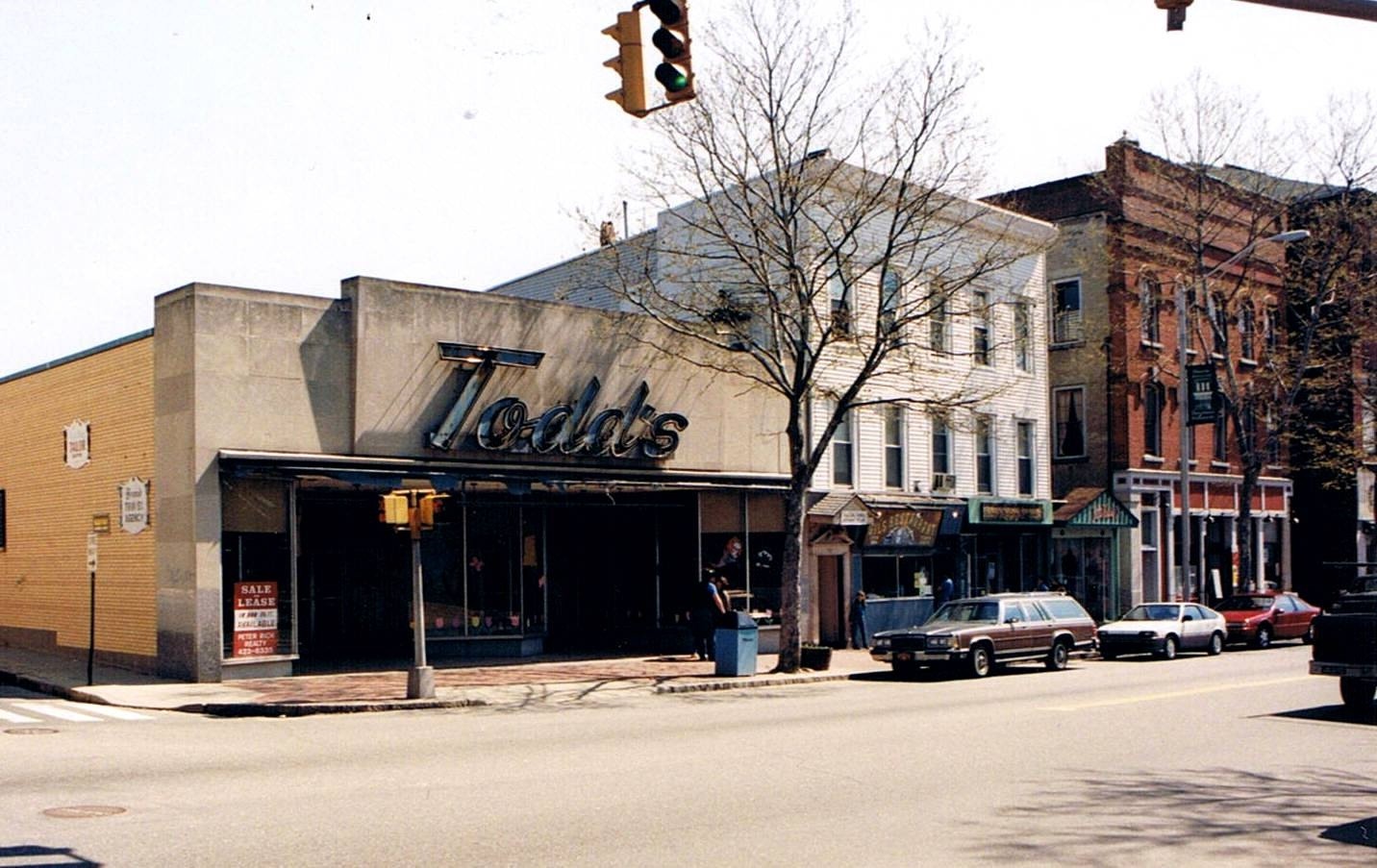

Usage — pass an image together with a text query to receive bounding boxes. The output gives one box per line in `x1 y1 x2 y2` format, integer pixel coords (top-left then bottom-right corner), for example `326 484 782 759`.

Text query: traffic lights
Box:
601 11 646 113
649 0 694 101
420 495 455 526
378 494 409 524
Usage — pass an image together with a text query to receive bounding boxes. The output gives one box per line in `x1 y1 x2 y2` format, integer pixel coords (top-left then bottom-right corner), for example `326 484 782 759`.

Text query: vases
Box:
801 643 833 672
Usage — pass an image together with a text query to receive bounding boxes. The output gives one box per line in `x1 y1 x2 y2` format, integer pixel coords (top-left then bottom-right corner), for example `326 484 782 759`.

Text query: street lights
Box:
1178 229 1311 602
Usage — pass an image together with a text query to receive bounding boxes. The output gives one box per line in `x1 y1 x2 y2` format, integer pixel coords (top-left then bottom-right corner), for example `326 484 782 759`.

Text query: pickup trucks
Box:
1309 561 1377 712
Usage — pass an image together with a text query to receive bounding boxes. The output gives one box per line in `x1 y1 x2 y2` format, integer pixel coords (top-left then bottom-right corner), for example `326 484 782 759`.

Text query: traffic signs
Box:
86 534 97 572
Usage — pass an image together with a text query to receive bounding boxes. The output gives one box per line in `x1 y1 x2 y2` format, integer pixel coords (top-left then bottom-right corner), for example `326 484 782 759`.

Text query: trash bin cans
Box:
714 611 759 676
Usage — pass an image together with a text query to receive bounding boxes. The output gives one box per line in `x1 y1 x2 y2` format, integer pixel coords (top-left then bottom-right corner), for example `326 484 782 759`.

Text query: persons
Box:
687 568 730 662
849 590 869 650
1039 577 1066 592
936 573 954 609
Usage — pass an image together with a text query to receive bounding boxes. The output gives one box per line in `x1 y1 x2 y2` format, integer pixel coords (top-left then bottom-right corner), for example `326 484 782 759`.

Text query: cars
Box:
1213 592 1324 650
1096 602 1228 661
869 591 1100 679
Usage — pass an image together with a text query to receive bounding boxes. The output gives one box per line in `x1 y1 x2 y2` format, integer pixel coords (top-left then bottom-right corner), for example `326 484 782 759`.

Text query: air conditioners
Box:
931 472 957 491
